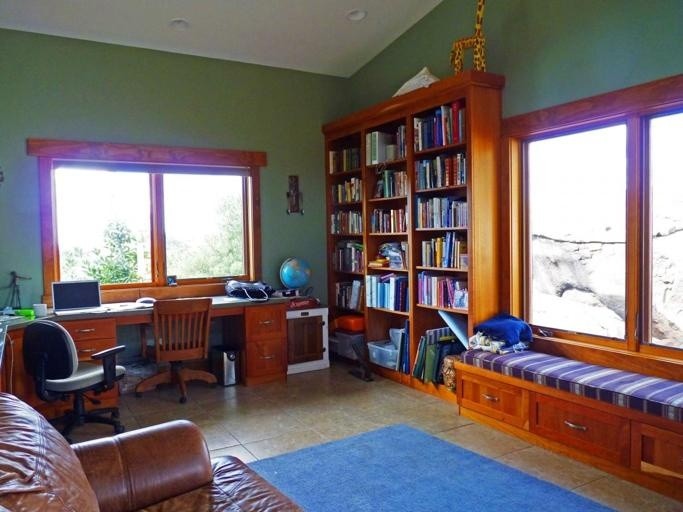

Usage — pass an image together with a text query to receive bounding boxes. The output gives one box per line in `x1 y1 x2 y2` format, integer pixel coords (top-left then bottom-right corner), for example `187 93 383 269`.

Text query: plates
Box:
14 310 33 316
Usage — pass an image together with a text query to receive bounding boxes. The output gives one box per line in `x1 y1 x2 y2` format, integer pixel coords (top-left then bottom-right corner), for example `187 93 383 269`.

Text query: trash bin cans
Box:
207 345 239 387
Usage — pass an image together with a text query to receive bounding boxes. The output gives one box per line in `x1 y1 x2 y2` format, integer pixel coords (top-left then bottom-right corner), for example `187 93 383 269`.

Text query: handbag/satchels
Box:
226 279 273 302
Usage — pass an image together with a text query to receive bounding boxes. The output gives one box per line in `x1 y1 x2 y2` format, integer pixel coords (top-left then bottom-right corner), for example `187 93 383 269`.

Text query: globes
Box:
279 256 312 299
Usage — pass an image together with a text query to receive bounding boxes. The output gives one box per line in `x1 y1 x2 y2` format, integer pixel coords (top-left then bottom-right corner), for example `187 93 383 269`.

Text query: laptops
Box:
52 280 107 316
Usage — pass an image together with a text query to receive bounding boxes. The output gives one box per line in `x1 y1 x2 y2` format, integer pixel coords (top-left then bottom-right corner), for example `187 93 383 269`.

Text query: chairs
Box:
21 319 126 444
135 298 217 403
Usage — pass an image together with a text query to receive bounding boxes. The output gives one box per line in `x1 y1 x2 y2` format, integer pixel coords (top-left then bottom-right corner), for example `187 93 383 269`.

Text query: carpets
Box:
244 423 618 512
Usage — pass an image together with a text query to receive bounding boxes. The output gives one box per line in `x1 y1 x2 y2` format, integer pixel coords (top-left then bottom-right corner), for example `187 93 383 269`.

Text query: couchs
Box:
0 391 303 512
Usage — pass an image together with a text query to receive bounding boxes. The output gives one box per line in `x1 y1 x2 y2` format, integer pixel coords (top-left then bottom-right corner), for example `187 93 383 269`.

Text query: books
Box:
329 101 468 384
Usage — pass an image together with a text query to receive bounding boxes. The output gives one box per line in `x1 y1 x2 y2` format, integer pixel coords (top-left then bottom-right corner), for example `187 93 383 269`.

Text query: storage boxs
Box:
366 340 398 370
334 331 363 360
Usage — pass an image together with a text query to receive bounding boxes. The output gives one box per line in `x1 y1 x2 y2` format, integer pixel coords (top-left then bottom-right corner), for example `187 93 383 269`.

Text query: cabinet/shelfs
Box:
454 362 683 505
7 327 56 417
222 304 287 386
287 308 330 374
56 320 118 414
322 68 503 406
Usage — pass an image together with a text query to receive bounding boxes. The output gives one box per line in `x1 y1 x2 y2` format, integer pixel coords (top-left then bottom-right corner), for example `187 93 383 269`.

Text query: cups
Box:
31 304 46 317
384 144 398 161
167 276 176 287
